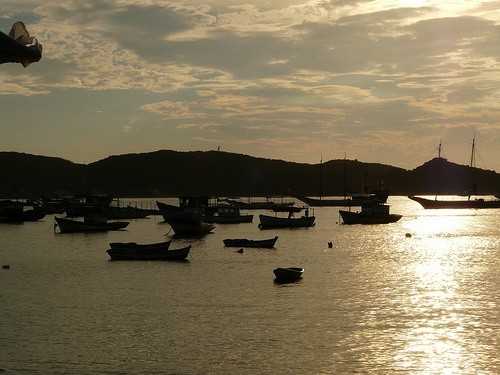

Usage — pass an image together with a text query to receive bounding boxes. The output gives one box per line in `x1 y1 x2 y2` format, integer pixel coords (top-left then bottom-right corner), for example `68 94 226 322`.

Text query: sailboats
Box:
406 132 500 208
290 153 391 206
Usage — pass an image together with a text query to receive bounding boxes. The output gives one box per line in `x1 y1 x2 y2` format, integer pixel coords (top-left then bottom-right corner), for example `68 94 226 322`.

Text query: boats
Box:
258 208 315 230
0 189 304 239
338 202 404 224
106 245 191 259
272 266 304 277
223 236 278 248
108 239 173 250
